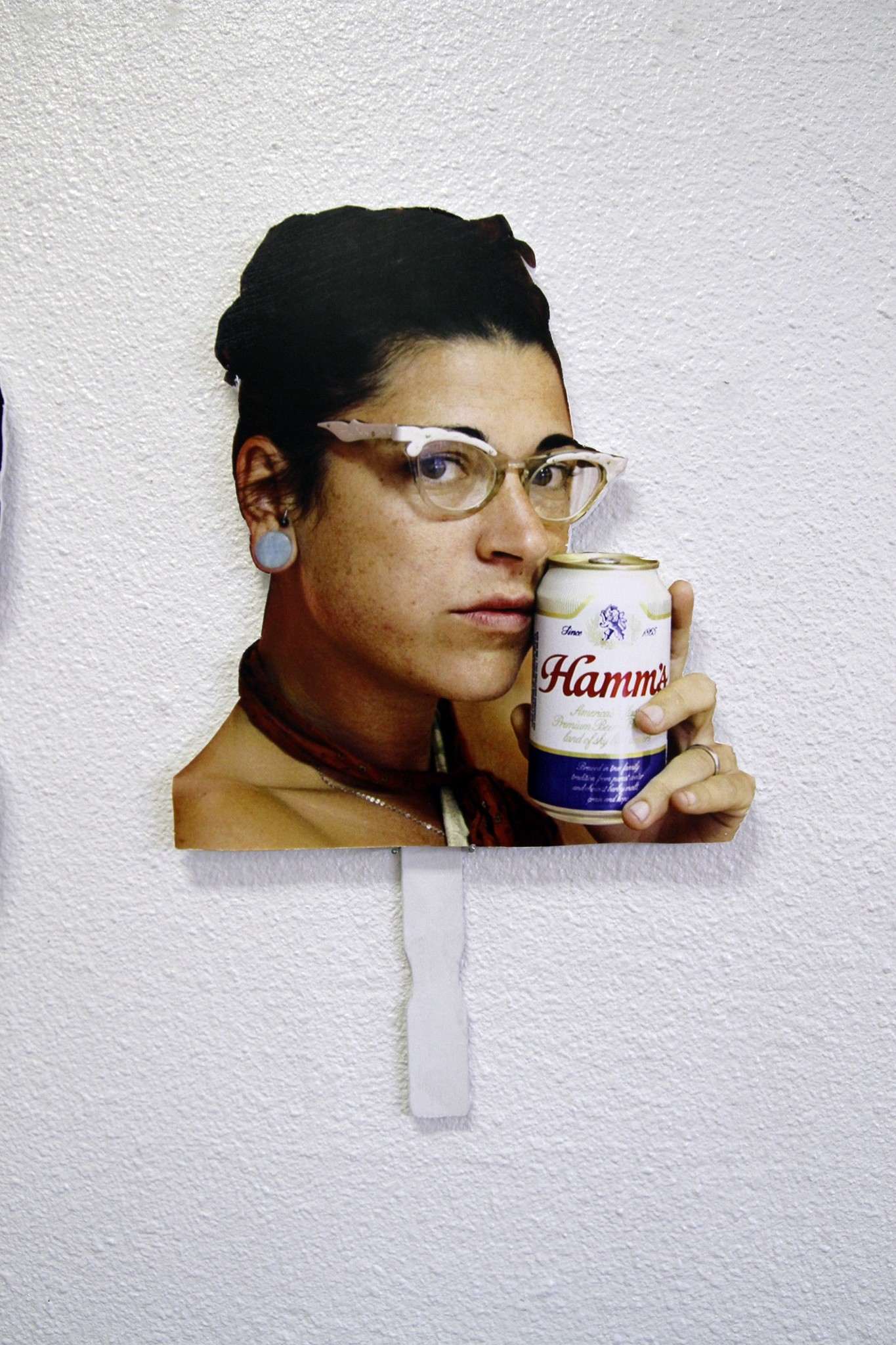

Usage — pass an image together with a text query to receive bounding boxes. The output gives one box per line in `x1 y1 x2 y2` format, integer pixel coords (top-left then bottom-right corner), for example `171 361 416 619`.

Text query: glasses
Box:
294 419 631 525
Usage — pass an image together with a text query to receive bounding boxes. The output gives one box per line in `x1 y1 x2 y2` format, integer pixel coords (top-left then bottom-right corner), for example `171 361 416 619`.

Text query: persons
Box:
169 205 753 848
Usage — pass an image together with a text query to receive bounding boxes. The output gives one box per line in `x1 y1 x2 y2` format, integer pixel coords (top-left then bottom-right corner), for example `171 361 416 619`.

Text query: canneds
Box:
523 554 678 833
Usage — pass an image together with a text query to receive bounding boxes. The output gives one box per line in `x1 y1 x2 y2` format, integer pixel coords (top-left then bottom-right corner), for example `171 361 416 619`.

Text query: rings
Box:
686 744 721 776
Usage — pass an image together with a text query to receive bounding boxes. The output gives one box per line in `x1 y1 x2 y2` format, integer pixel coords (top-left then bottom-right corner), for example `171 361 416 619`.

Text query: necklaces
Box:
315 767 487 847
